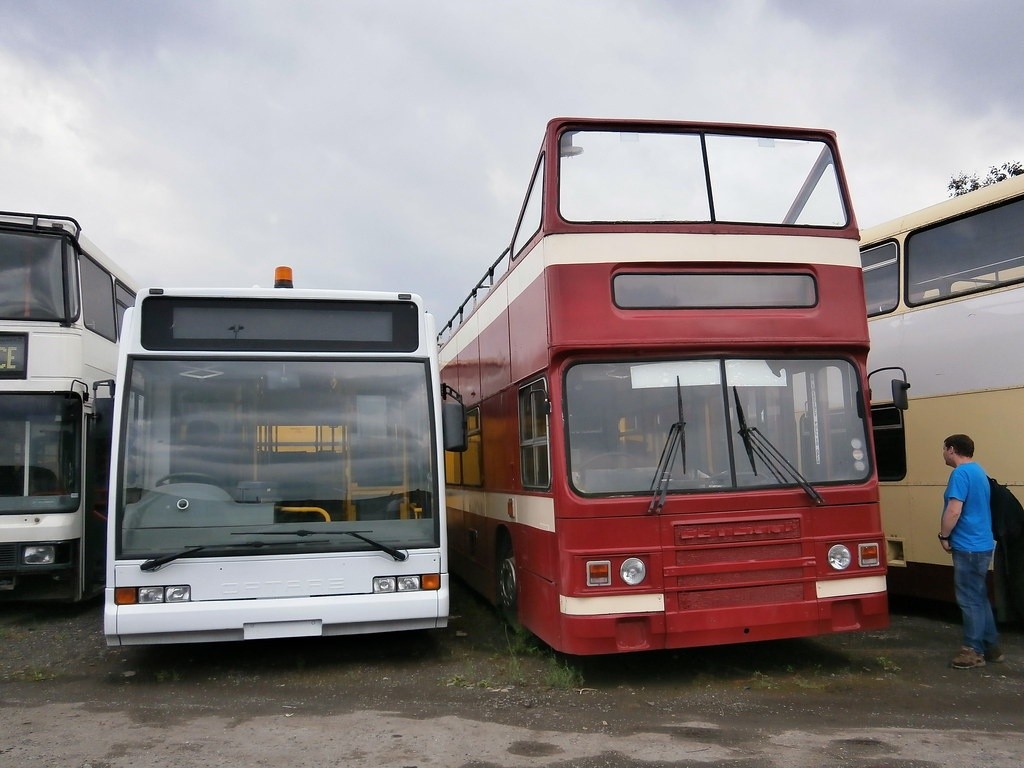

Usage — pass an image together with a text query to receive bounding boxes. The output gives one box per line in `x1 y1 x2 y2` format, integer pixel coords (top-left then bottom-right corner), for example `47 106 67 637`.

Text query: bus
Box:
0 111 1021 680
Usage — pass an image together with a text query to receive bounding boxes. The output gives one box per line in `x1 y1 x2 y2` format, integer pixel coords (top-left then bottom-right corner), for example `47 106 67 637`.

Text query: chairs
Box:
169 419 425 520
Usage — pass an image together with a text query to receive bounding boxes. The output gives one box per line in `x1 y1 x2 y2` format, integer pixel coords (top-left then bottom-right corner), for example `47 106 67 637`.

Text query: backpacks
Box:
986 474 1024 544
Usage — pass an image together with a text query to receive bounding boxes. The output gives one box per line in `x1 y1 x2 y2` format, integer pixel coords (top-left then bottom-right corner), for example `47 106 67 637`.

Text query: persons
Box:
934 433 1004 669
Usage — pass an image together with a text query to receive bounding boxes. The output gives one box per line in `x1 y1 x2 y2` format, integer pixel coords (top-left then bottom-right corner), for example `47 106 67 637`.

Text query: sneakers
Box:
952 649 986 669
985 648 1003 662
